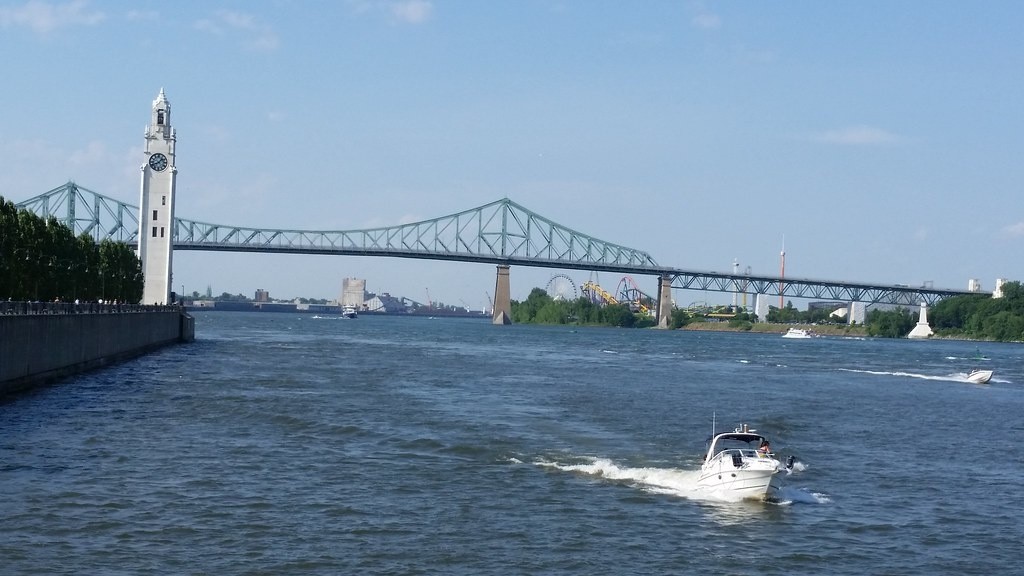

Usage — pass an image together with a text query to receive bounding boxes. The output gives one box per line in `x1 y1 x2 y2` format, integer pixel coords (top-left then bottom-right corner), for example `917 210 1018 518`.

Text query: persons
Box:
757 441 773 458
6 296 117 314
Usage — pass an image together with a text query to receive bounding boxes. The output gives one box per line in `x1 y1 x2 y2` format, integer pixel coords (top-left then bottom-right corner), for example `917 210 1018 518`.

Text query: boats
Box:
341 303 358 318
782 328 814 340
967 369 996 384
696 412 796 502
